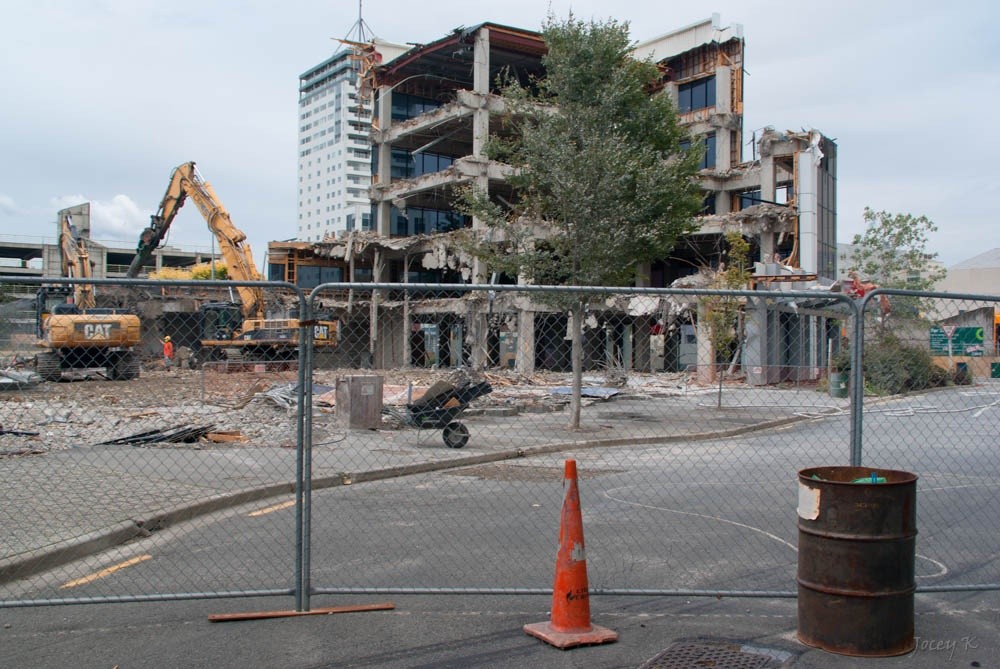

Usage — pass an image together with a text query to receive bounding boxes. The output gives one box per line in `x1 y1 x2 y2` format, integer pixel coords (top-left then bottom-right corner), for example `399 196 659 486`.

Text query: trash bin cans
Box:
991 362 1000 378
797 466 919 657
831 367 849 398
956 361 972 385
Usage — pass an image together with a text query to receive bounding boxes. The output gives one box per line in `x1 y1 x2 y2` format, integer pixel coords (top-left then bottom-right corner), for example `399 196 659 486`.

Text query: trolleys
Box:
405 380 495 449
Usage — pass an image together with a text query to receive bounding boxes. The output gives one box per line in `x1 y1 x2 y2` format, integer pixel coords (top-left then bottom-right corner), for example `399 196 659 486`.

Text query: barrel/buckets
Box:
798 466 919 658
830 372 848 399
991 362 1000 378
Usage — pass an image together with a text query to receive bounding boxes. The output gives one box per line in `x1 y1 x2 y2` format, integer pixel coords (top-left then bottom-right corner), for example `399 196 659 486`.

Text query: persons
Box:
160 336 173 372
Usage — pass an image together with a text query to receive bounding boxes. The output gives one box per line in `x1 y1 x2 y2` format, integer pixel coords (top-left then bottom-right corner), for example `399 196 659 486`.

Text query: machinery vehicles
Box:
120 160 341 373
32 209 142 384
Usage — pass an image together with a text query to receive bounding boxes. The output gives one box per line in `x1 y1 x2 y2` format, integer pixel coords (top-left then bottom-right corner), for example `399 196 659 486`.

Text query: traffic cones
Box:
519 457 619 650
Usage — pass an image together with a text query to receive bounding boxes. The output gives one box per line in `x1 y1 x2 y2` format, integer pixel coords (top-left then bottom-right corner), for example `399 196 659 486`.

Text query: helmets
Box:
164 336 170 340
